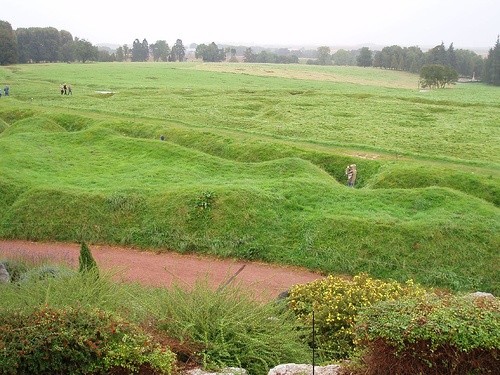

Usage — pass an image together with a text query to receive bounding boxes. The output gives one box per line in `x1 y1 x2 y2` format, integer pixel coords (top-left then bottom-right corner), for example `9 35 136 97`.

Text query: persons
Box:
60 87 64 94
345 164 356 189
0 88 3 98
4 85 10 96
160 134 164 140
68 84 73 95
64 83 68 94
